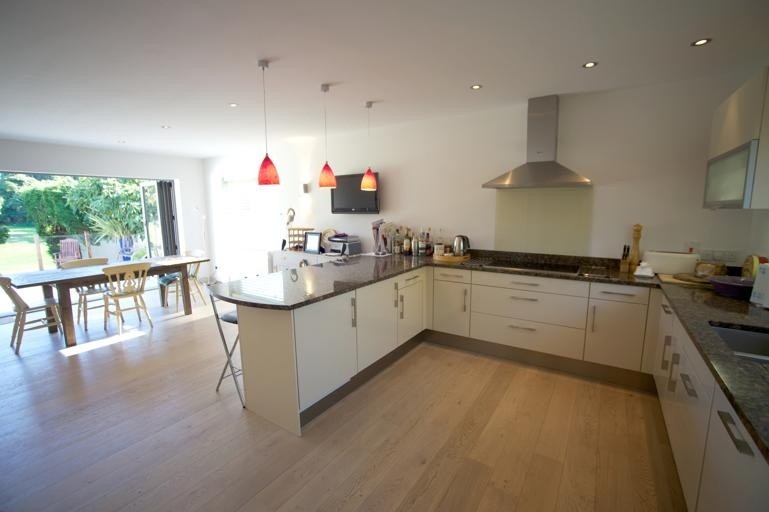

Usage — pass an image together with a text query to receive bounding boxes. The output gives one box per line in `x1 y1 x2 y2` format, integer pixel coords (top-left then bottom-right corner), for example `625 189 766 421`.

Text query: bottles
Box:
393 228 432 256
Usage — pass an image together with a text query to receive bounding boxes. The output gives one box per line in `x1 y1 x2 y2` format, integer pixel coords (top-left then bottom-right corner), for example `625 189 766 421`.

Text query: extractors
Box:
482 95 592 189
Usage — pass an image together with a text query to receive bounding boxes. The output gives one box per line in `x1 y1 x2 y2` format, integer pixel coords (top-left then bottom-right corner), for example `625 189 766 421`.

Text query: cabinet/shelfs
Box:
268 251 342 274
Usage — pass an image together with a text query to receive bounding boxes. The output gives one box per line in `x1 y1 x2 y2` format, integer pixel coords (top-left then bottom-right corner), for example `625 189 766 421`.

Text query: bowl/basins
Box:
707 275 757 298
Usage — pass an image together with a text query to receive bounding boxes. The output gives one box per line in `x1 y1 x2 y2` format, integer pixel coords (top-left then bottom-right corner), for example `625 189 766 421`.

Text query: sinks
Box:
709 320 769 360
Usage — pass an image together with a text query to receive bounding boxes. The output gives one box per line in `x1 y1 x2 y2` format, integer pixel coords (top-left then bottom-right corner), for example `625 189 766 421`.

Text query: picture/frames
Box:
304 231 322 254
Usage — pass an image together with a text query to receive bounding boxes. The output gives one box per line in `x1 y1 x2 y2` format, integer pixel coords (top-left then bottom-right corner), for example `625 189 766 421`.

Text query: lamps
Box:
258 59 280 186
361 102 377 192
318 83 337 189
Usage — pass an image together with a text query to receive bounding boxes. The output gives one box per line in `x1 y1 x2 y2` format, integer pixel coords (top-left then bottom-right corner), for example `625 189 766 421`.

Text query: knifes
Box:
622 245 630 260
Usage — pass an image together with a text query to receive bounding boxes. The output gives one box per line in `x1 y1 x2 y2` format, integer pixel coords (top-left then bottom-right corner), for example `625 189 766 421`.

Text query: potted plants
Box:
89 210 134 262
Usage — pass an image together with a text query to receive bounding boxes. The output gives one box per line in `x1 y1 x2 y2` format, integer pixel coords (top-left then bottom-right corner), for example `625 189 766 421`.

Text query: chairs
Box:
210 294 245 408
51 239 80 263
164 261 207 312
0 276 64 354
102 263 154 335
63 258 125 331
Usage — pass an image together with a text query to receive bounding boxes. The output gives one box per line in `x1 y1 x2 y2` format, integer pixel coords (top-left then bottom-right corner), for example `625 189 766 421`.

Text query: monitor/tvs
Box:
331 172 380 214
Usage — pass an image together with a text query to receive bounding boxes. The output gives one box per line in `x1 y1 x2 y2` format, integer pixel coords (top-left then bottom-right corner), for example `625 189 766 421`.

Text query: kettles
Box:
453 235 470 256
